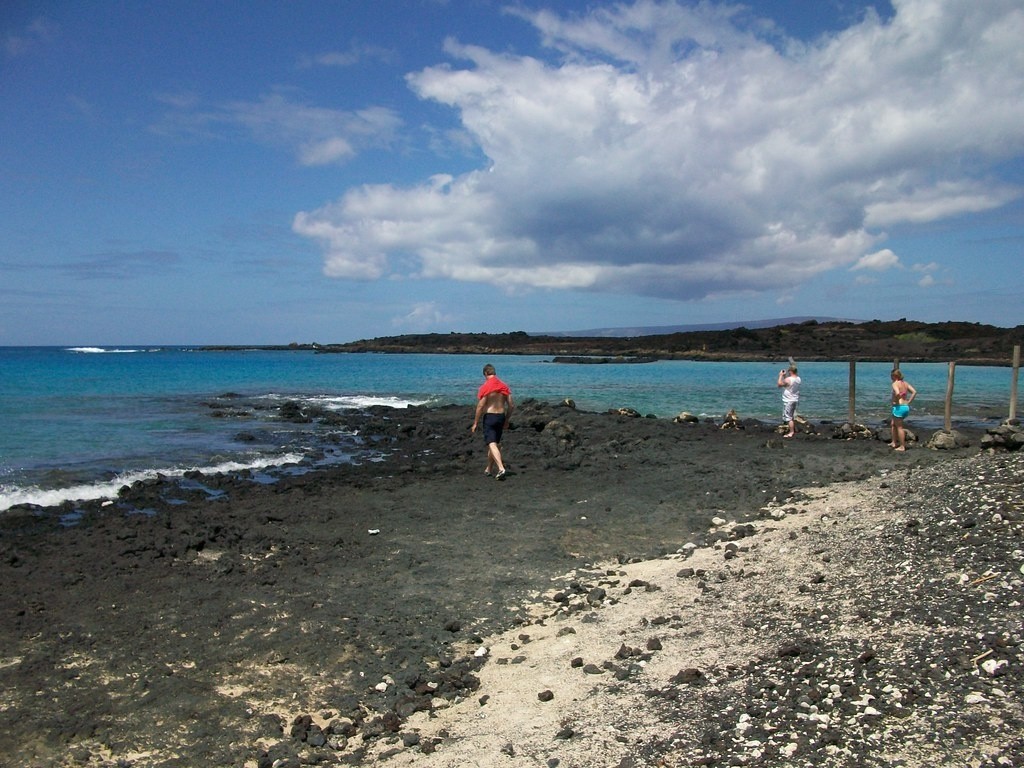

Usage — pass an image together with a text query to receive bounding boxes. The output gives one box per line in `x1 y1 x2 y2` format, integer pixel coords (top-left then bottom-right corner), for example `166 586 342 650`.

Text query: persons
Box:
778 366 801 438
472 364 513 479
887 369 916 451
